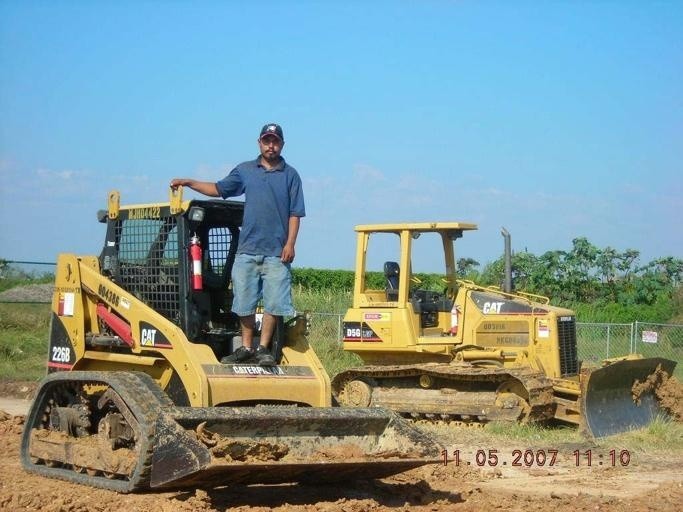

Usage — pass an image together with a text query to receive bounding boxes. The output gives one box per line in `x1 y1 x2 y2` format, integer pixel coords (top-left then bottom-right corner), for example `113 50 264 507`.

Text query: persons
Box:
167 122 305 366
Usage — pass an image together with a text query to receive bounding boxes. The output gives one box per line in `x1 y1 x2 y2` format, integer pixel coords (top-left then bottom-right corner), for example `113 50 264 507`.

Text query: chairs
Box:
381 261 439 328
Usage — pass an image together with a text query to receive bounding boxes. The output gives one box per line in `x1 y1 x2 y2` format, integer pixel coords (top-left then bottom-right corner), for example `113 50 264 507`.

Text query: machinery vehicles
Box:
329 222 677 442
18 185 454 494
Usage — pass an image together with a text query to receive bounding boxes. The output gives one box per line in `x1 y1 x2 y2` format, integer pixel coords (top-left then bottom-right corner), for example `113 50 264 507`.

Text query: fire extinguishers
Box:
450 304 460 336
187 231 205 292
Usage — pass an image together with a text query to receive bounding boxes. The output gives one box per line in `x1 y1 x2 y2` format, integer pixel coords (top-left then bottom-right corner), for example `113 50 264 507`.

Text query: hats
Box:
259 125 283 142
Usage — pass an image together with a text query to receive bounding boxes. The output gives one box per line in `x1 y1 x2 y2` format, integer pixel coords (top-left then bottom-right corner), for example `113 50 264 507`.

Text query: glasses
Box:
262 139 280 145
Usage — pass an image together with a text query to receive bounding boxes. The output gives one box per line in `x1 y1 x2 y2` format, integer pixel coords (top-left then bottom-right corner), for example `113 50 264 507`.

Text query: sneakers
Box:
221 346 255 364
256 345 274 366
